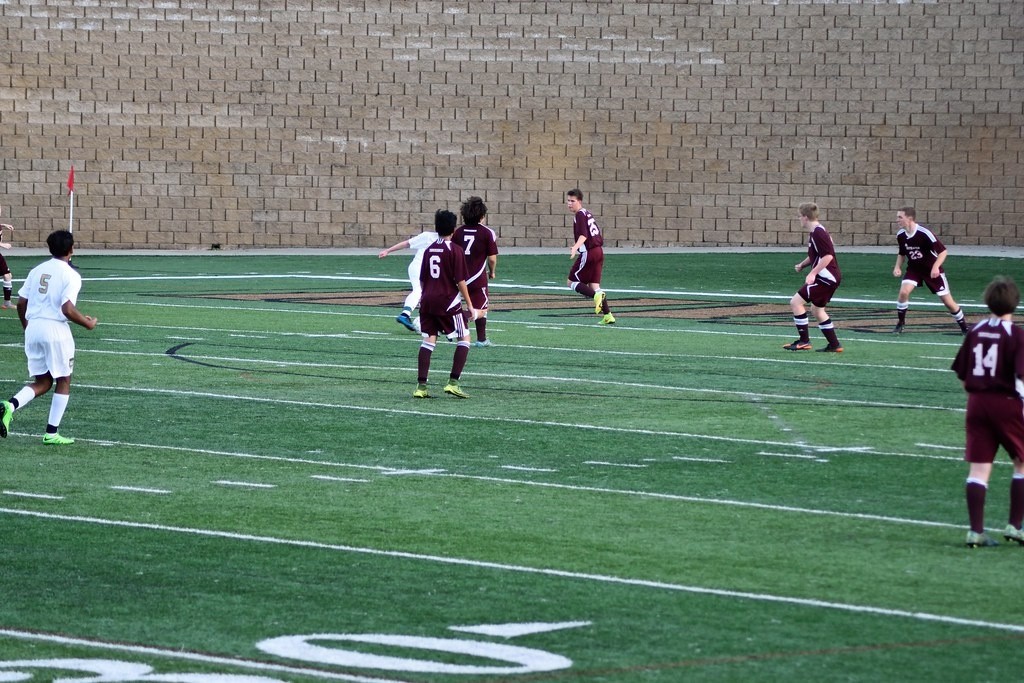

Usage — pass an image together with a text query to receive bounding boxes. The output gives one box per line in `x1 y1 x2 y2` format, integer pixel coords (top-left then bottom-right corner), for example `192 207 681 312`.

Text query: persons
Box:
413 209 475 397
452 196 498 348
0 224 17 310
0 230 98 445
950 283 1023 548
782 202 843 352
893 206 970 335
379 231 438 335
567 189 615 325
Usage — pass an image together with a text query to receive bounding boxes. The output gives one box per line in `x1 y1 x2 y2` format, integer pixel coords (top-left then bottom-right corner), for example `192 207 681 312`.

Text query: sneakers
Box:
2 305 17 309
597 311 616 324
593 292 605 314
893 322 905 335
966 530 1000 548
815 343 843 351
1003 524 1024 546
0 401 13 438
413 383 438 397
963 327 967 338
396 314 416 330
413 316 421 334
475 339 490 346
42 428 74 445
445 379 470 398
783 339 812 350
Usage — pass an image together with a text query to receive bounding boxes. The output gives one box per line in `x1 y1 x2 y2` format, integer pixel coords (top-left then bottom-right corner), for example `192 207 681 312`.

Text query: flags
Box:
66 166 74 196
